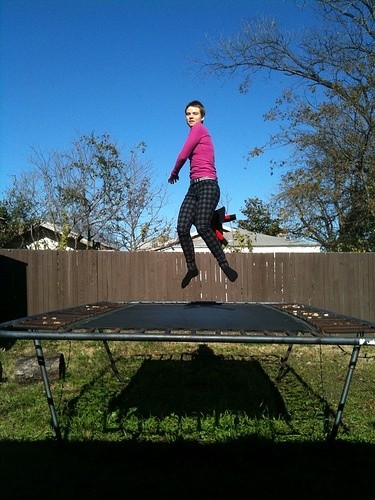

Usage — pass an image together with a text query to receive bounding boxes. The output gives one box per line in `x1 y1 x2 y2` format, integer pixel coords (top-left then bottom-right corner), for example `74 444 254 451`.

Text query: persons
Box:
167 100 238 289
